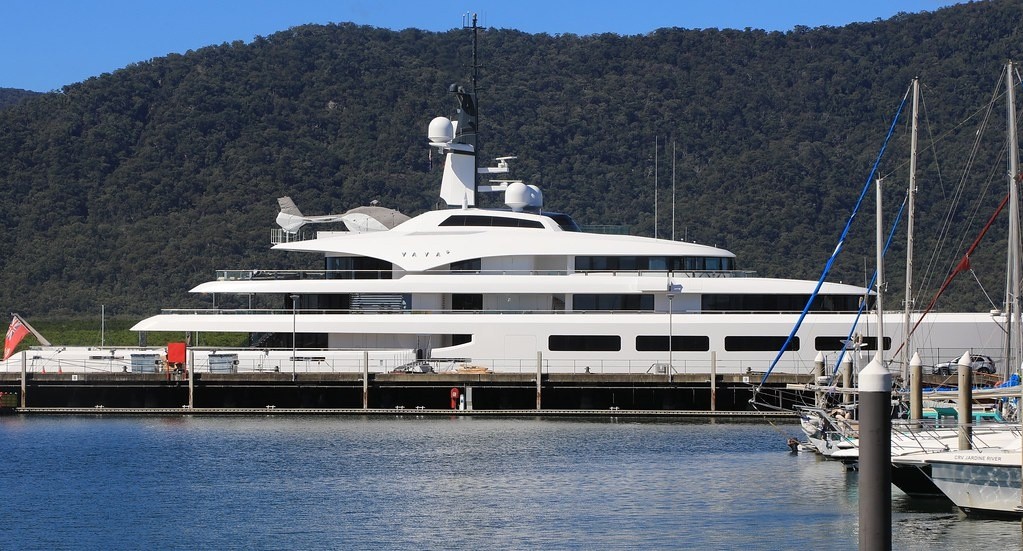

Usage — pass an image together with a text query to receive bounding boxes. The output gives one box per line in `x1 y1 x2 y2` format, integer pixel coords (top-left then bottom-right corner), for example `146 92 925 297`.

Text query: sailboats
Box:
746 57 1022 519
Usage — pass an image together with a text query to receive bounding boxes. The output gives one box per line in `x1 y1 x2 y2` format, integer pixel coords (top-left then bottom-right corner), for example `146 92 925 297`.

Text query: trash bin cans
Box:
654 363 669 374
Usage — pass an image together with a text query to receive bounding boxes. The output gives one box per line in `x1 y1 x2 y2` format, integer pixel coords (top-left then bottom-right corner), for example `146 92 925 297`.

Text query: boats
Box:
0 13 1022 410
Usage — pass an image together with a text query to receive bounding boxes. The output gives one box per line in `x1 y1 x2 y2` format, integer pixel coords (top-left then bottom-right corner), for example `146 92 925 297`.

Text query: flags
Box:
3 316 30 362
